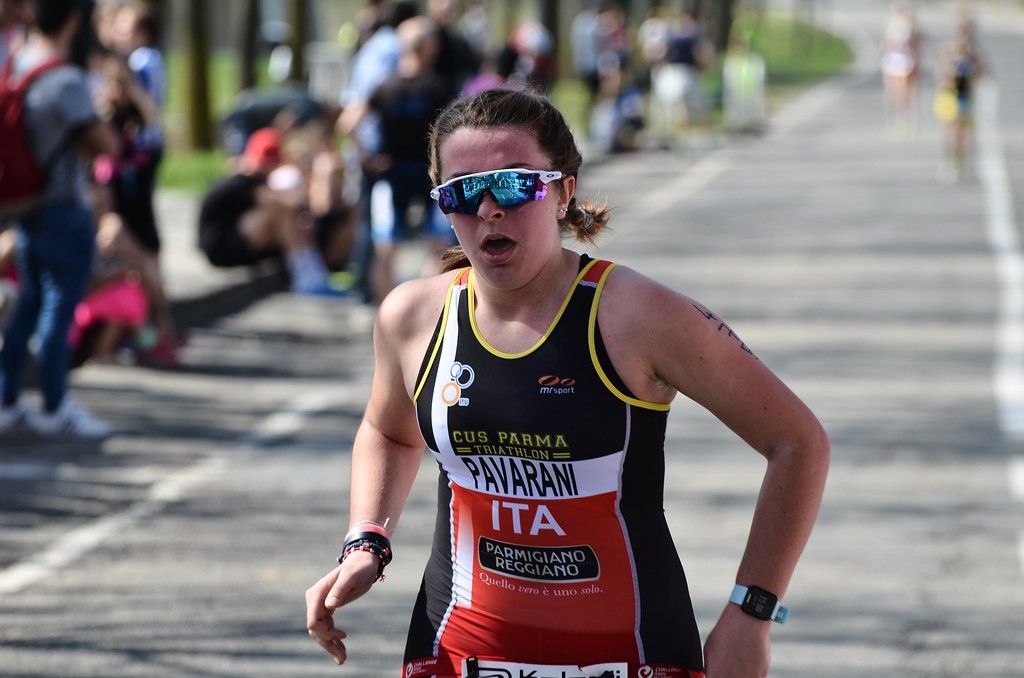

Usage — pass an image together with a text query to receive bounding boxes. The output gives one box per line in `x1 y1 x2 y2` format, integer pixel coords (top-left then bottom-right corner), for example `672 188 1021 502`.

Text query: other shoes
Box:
30 395 110 440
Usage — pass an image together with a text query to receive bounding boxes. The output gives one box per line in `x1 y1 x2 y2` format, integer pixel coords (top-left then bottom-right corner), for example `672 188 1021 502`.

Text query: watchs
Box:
730 584 789 624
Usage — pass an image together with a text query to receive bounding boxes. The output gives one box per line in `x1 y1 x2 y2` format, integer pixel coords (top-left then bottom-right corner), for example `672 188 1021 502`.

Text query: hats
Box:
245 127 283 165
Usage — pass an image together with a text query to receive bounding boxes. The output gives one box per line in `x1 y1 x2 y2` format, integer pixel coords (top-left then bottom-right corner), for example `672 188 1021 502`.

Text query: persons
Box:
0 1 726 474
306 90 835 678
880 6 924 116
936 16 991 185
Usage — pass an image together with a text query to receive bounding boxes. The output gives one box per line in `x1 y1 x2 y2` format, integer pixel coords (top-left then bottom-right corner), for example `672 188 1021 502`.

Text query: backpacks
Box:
1 56 67 210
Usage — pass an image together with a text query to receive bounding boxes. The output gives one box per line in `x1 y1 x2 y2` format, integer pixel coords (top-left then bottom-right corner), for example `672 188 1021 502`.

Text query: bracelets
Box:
337 521 393 584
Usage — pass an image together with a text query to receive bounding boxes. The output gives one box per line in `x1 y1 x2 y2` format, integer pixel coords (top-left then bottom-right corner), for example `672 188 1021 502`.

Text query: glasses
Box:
429 168 568 214
